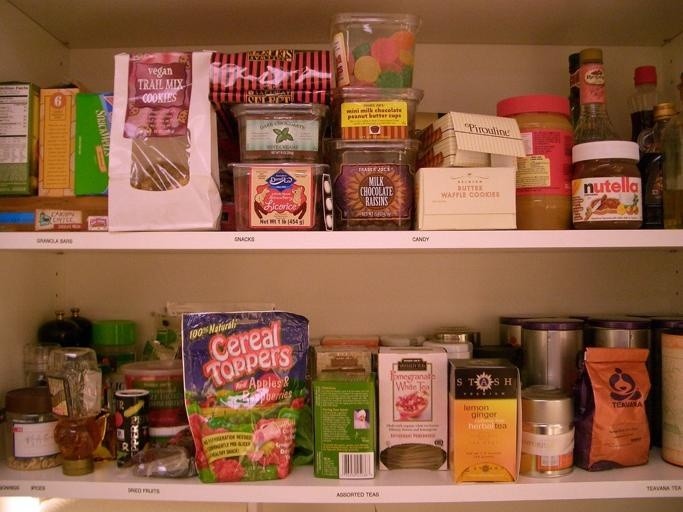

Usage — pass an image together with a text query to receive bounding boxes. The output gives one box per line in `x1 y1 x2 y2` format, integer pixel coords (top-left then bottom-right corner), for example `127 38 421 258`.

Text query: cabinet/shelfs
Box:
0 225 681 511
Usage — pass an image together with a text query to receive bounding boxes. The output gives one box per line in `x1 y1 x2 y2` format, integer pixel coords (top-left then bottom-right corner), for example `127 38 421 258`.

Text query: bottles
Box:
89 319 137 407
47 346 103 476
568 48 619 143
625 66 683 230
21 307 92 391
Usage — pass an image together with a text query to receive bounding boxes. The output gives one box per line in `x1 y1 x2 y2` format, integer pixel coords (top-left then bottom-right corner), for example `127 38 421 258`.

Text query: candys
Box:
334 12 417 89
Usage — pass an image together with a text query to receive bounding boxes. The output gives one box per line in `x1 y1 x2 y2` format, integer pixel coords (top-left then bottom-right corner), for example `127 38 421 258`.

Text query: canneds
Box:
498 94 575 230
3 388 61 470
497 315 683 478
115 389 150 467
570 139 645 230
